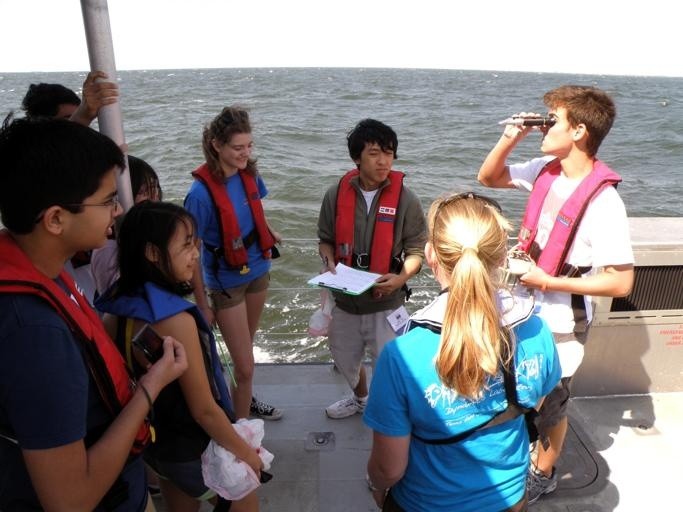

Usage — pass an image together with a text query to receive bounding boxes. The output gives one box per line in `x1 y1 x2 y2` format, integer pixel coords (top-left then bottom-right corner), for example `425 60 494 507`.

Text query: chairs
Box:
526 462 558 506
145 484 162 501
324 393 368 419
249 395 284 421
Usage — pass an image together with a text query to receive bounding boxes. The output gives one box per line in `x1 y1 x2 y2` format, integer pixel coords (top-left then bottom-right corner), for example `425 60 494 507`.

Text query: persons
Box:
180 105 282 421
0 113 189 510
363 191 567 511
19 69 119 127
91 153 161 296
99 198 265 511
476 82 636 506
317 118 427 421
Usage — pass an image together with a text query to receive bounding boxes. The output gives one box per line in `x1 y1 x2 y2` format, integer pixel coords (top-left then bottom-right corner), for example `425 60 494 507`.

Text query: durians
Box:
499 117 555 126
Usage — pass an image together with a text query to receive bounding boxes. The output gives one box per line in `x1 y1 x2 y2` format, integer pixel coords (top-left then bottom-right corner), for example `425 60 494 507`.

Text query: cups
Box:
83 193 120 213
431 190 505 243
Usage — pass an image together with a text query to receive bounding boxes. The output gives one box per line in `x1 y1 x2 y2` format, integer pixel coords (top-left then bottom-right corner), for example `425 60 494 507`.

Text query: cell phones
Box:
132 323 167 359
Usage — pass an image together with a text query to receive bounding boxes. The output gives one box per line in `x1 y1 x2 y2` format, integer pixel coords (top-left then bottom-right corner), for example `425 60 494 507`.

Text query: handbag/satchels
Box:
363 474 383 491
539 274 552 292
